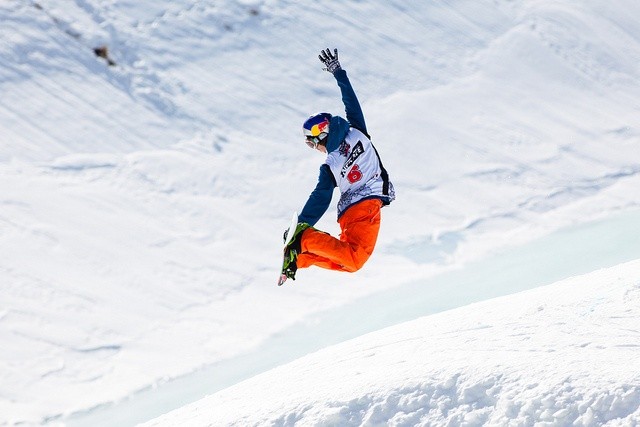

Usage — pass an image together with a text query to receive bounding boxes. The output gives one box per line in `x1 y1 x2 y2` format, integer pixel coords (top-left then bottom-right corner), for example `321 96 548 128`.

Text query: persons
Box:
282 48 395 280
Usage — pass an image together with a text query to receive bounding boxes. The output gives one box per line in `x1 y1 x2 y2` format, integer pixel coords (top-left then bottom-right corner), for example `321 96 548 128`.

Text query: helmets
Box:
303 113 332 136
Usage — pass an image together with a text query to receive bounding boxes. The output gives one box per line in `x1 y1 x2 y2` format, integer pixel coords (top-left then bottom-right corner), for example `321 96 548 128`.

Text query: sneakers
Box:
278 264 298 285
280 222 310 268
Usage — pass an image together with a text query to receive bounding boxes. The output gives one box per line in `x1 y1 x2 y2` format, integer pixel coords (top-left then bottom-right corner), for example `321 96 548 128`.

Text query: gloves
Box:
316 47 341 73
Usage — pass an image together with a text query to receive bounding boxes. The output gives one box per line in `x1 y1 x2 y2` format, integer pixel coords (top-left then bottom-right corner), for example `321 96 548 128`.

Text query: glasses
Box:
303 133 328 149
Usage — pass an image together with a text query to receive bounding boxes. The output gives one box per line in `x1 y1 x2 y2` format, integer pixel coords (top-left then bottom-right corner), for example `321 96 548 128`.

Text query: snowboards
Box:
278 212 297 286
